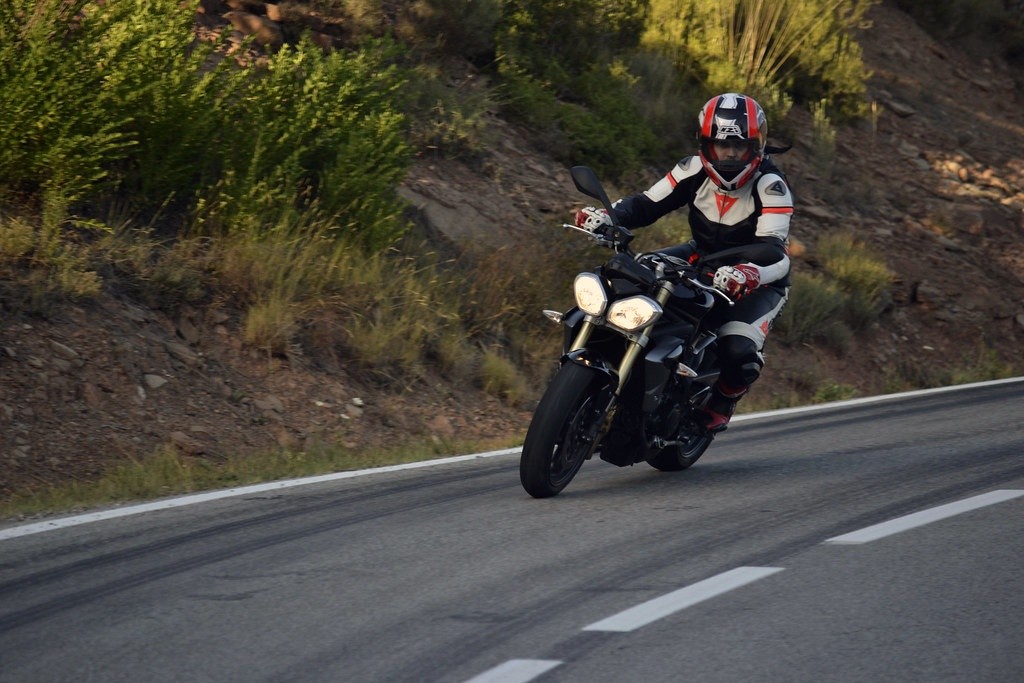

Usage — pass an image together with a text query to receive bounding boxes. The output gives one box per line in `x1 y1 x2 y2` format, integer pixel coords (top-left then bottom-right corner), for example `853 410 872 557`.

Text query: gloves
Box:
713 265 761 298
574 207 613 242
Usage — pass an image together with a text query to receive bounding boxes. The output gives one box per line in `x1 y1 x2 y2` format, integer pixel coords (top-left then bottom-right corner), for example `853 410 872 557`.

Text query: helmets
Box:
695 93 768 191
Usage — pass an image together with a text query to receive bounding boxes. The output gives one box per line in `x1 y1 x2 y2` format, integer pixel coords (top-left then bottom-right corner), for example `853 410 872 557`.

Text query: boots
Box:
700 381 749 431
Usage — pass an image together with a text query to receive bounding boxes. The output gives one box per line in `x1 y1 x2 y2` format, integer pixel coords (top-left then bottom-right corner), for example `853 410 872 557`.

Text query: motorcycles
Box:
520 166 784 498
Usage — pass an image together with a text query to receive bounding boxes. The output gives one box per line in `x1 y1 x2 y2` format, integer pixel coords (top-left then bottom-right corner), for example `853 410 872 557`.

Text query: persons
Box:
574 93 795 436
